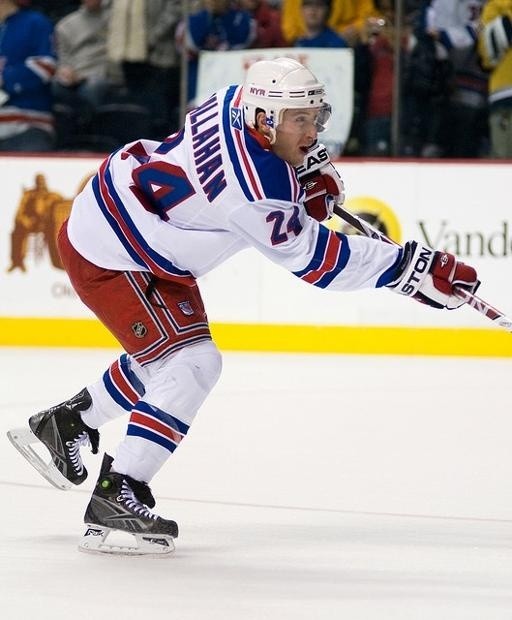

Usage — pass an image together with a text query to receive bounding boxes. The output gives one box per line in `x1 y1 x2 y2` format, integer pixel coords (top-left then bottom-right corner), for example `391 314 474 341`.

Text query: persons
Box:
29 56 478 535
0 0 512 160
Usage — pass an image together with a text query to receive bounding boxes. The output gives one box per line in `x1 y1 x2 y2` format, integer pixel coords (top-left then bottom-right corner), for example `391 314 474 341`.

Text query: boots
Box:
6 385 90 493
80 452 179 559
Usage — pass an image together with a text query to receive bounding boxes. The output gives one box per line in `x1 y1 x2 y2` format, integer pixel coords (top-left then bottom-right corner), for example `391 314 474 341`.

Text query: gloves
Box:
289 144 350 221
387 241 479 313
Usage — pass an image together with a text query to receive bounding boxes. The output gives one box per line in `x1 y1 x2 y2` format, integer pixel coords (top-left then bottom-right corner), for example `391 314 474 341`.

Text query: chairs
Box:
45 71 173 158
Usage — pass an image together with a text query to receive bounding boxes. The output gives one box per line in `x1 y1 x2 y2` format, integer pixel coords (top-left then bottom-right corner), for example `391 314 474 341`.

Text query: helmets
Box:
241 57 333 141
